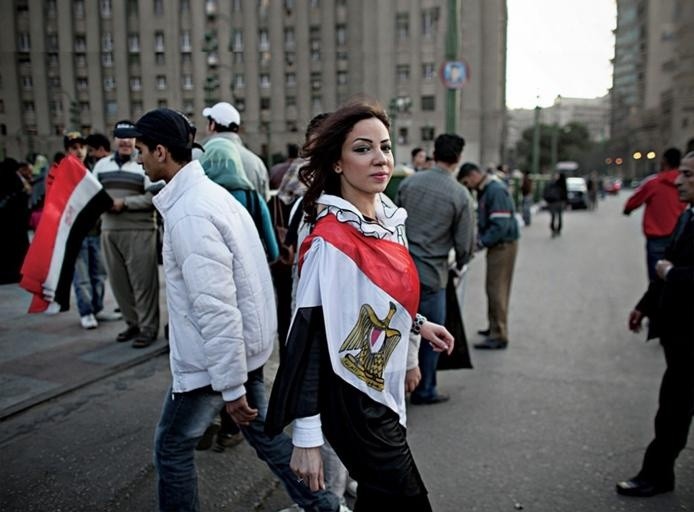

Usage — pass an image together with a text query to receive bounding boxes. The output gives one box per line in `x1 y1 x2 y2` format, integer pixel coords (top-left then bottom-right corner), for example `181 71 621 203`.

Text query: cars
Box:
566 177 588 210
604 176 621 195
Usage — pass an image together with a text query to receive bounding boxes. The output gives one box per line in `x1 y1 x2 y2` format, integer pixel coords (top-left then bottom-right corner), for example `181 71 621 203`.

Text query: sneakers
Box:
117 328 140 342
196 422 219 449
96 310 123 321
215 431 244 451
552 230 555 236
81 314 98 330
133 335 156 348
557 229 561 236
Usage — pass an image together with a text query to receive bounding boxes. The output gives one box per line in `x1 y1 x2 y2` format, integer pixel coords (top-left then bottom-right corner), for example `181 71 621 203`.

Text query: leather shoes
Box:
615 480 674 497
474 337 508 349
411 391 449 405
478 329 491 336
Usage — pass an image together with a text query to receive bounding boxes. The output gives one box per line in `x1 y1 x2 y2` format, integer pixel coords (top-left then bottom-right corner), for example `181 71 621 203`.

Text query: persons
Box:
1 118 162 349
196 100 334 349
261 102 458 512
613 152 692 498
543 171 565 238
132 107 348 512
619 144 684 319
391 134 535 405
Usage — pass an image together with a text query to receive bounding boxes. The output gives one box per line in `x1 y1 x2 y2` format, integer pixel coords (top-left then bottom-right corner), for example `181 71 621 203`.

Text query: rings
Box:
296 478 303 482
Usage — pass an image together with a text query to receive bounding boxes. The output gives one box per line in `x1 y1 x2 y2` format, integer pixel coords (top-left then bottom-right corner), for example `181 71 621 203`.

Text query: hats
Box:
202 102 241 127
63 132 87 150
115 120 135 129
112 109 205 151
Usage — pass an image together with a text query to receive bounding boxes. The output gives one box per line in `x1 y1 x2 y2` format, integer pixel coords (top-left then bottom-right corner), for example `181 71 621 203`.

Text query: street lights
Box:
633 151 655 179
